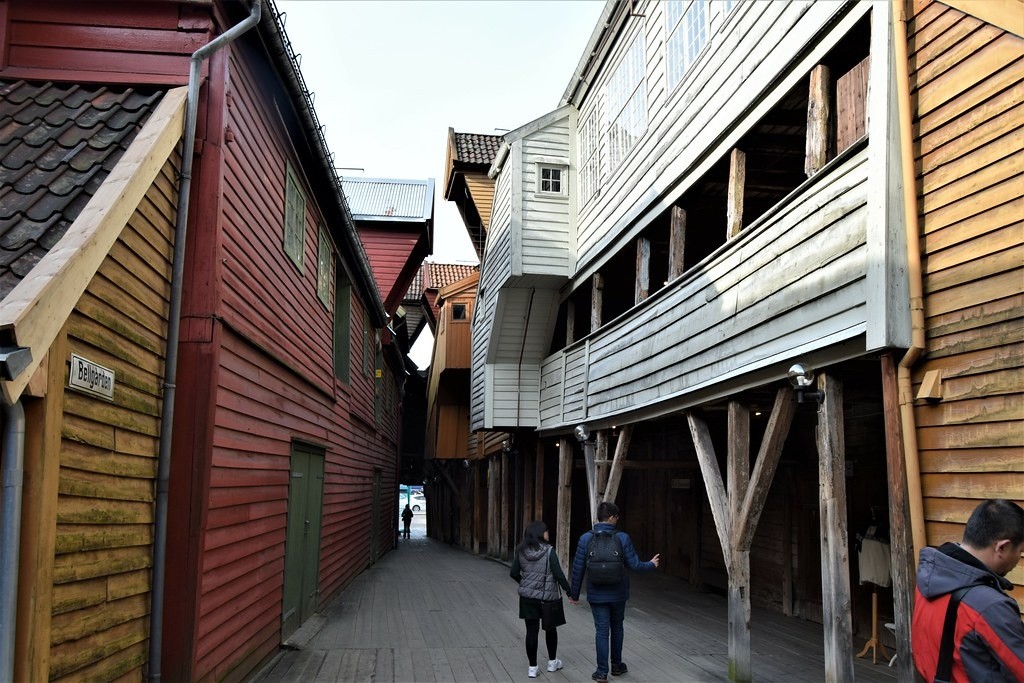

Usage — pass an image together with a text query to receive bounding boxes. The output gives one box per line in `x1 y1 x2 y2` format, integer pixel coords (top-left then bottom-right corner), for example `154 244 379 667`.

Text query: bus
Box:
400 485 412 498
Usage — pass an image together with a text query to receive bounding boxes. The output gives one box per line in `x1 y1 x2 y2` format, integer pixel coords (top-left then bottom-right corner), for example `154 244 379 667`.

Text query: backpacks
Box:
584 528 630 586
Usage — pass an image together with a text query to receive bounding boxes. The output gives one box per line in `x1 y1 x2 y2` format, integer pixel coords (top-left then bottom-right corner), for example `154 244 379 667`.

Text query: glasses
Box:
614 515 619 519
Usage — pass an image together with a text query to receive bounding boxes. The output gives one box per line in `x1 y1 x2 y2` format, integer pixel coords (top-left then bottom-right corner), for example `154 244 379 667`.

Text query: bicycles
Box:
402 514 413 539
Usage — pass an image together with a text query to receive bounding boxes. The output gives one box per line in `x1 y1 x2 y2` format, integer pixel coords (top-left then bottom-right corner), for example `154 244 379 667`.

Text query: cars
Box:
411 489 421 495
403 494 426 512
399 493 408 509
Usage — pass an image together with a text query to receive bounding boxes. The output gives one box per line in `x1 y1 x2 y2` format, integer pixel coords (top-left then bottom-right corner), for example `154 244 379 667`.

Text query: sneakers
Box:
547 657 563 672
610 662 629 675
527 665 541 678
591 671 607 683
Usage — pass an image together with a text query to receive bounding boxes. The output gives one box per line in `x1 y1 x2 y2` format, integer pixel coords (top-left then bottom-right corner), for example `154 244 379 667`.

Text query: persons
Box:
569 503 660 682
509 522 575 679
903 497 1024 683
401 504 413 540
